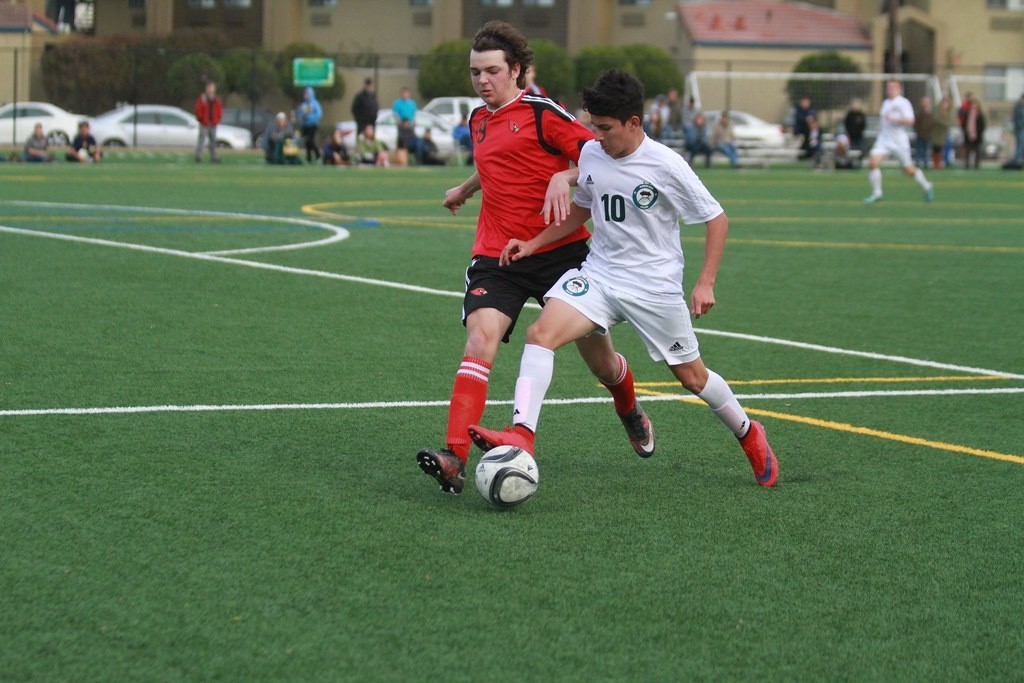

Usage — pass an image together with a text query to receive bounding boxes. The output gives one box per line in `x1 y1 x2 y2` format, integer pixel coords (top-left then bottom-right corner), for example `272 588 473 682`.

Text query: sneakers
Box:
467 424 535 458
417 448 467 493
737 420 779 487
615 400 656 459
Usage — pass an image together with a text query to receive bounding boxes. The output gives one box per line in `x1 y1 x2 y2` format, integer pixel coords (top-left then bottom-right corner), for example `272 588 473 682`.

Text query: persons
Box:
297 87 323 164
711 112 740 167
67 122 103 163
644 88 711 166
466 71 778 488
914 97 954 170
25 124 56 162
323 129 353 165
352 79 379 133
1013 93 1024 164
452 114 474 165
265 110 302 165
194 82 221 162
415 20 655 496
862 80 935 203
355 125 390 167
392 88 447 165
959 93 985 167
782 98 864 168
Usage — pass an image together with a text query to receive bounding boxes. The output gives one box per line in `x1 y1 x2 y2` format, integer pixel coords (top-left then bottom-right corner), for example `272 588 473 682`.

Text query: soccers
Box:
474 444 540 505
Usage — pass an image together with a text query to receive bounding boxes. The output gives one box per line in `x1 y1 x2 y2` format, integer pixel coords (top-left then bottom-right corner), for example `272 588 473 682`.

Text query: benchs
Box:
660 134 784 170
443 136 472 168
853 132 918 170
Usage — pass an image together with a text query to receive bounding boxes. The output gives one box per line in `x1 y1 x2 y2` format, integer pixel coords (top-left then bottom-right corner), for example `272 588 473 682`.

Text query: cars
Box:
81 103 252 149
830 112 1006 161
425 97 481 128
334 108 458 163
0 100 93 147
703 110 786 152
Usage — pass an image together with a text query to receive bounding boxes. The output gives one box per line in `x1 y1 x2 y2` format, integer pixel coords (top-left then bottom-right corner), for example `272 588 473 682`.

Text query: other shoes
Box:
925 183 934 201
865 193 883 205
